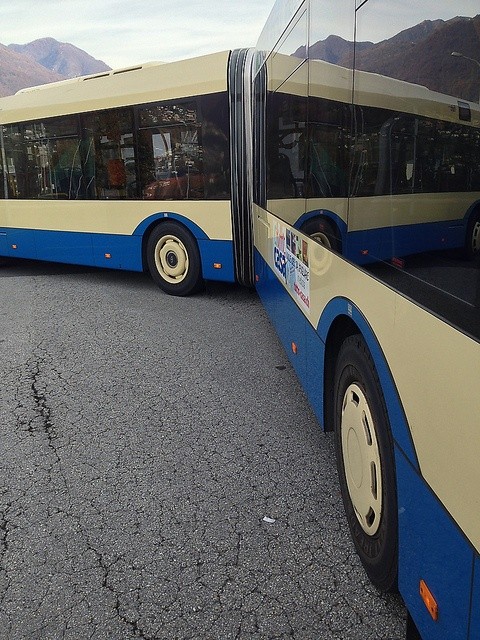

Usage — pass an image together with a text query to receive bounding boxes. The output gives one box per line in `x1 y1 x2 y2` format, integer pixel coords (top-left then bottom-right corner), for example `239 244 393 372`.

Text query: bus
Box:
0 0 480 640
262 50 480 267
